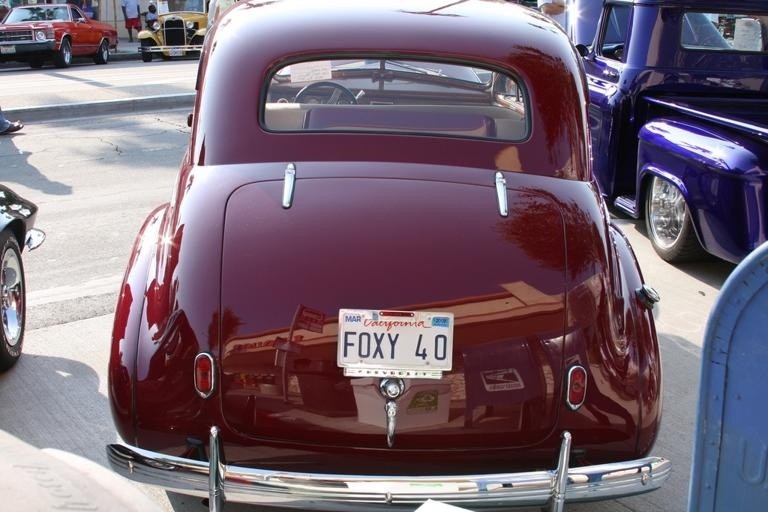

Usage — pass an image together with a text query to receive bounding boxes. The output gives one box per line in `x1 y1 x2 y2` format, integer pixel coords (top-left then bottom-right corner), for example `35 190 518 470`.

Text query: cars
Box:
109 0 670 510
0 5 116 67
0 181 44 361
138 0 207 62
571 0 766 265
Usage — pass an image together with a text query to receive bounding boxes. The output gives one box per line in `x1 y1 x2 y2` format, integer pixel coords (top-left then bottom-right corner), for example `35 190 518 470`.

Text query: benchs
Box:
266 101 518 139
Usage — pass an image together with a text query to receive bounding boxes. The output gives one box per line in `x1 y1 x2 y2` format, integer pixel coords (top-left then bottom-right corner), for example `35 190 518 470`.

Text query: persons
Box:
537 0 567 35
120 0 142 42
0 103 26 136
755 14 768 51
144 4 158 30
702 12 719 30
